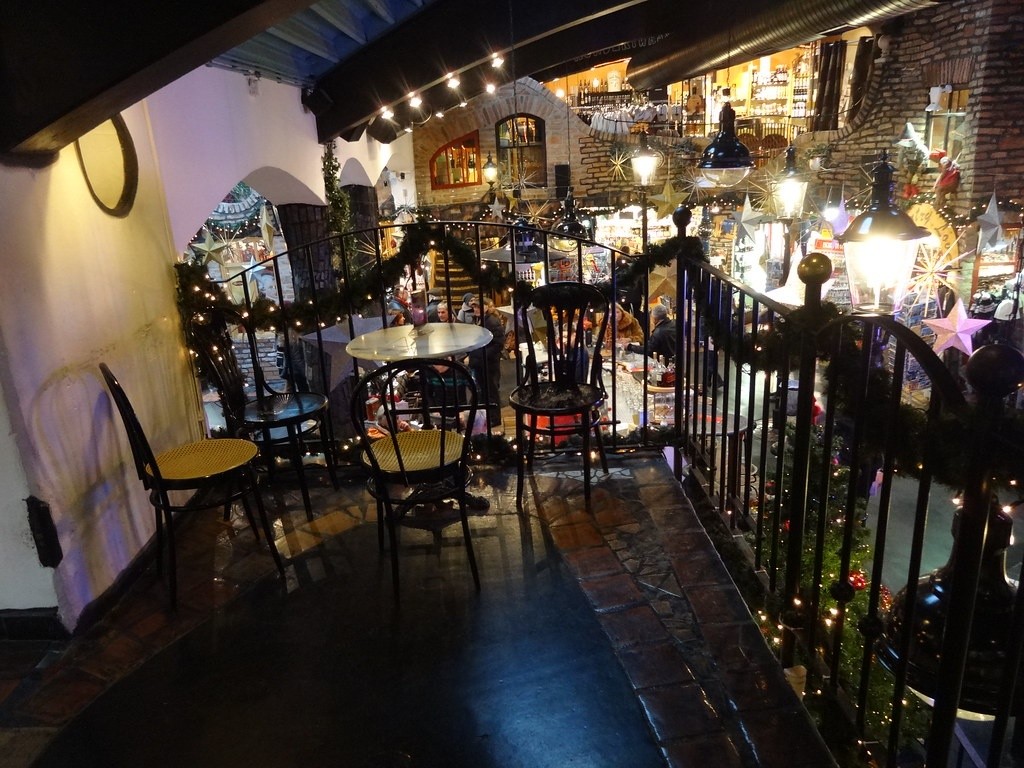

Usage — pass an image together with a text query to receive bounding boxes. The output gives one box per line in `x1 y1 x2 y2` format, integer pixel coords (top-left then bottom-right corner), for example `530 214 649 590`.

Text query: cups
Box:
624 351 635 361
632 410 639 425
639 410 654 428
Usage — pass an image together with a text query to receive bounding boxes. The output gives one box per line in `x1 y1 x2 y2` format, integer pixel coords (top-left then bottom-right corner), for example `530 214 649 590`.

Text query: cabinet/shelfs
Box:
716 75 809 118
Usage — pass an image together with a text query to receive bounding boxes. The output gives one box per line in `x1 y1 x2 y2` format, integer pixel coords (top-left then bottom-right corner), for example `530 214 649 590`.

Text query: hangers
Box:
577 100 680 121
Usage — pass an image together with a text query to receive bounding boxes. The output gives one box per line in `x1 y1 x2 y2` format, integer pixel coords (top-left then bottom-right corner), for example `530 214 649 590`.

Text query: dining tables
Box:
495 306 694 435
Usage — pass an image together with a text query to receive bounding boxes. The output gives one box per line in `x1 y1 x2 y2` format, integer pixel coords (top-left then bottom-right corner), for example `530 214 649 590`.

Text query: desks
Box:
345 319 495 523
677 404 756 436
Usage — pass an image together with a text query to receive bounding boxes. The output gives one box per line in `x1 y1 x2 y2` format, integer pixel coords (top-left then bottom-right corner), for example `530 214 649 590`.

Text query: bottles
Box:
650 352 678 387
586 325 592 347
616 345 623 359
656 389 694 418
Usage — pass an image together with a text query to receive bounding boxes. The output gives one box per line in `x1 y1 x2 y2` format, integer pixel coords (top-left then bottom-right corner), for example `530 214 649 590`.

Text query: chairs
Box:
350 356 480 613
187 306 340 524
99 360 285 623
509 280 609 514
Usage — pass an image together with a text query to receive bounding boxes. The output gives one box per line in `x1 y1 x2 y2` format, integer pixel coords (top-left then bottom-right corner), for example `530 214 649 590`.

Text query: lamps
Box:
480 0 566 273
837 144 931 315
697 22 754 188
772 141 811 220
549 187 588 250
925 85 953 112
630 121 659 186
483 151 499 208
881 496 1021 722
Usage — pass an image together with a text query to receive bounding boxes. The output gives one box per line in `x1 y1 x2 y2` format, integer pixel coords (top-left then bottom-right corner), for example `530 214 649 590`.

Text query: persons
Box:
702 335 726 389
377 403 410 433
426 288 443 322
429 300 465 418
389 310 406 326
389 285 413 324
892 122 929 199
553 323 589 384
598 302 644 349
454 293 476 365
930 149 960 206
623 305 677 370
685 87 702 137
474 297 506 428
